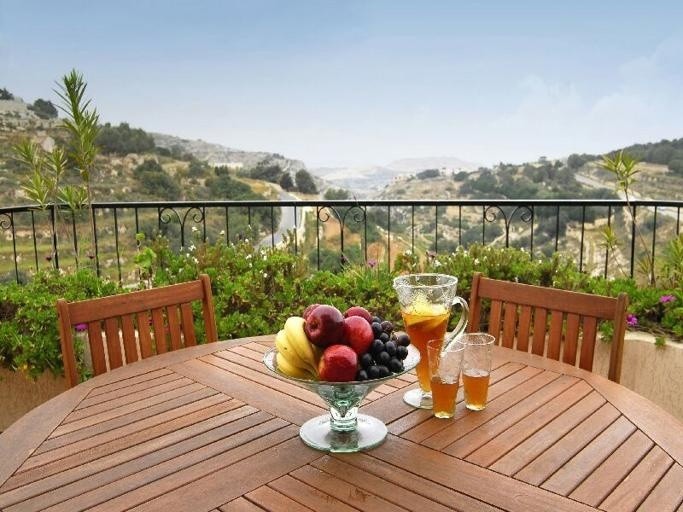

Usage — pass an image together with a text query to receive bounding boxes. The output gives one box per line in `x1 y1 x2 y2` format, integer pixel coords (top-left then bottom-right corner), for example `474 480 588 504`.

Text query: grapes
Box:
356 317 410 378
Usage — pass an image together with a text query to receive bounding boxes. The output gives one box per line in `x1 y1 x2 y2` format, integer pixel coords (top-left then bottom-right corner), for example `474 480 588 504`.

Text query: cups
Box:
425 339 464 420
459 333 495 410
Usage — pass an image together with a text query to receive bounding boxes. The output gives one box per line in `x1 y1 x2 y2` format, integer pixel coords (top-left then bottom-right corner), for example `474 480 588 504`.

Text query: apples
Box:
300 303 373 380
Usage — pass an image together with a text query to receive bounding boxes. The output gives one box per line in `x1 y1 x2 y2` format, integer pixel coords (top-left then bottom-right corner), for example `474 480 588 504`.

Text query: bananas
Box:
275 316 318 381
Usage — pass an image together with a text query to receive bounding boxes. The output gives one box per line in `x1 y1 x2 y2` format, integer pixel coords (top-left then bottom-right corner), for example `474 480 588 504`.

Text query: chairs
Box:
56 274 219 390
468 272 628 384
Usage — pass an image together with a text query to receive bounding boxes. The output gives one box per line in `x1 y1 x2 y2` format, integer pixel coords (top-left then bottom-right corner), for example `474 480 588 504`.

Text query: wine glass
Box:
391 272 470 409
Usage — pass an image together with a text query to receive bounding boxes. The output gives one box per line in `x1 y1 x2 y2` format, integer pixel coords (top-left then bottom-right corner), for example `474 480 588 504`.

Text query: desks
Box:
0 334 683 512
